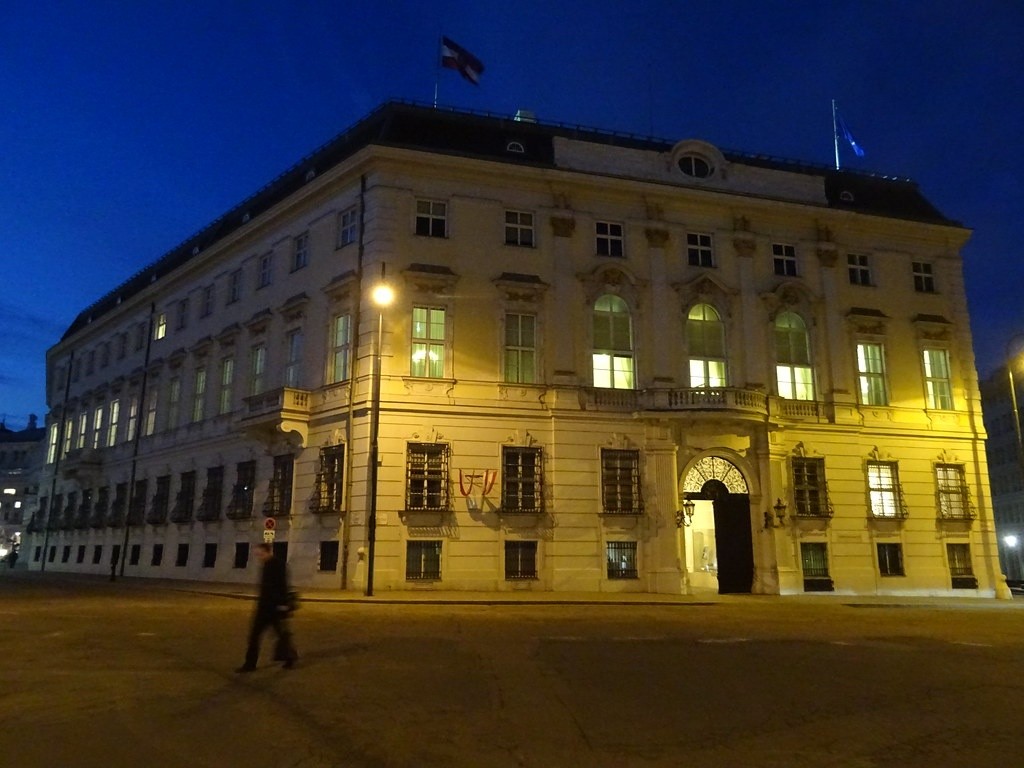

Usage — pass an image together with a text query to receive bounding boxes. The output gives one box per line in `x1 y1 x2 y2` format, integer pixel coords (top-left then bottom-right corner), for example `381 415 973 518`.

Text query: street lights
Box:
368 284 395 594
1004 533 1022 585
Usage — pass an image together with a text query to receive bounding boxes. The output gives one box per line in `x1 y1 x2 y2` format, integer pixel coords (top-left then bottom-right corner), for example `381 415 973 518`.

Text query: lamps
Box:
764 498 786 530
676 496 695 528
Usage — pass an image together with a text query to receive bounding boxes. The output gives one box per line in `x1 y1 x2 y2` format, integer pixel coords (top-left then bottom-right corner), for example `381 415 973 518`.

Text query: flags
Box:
838 115 864 158
442 37 484 83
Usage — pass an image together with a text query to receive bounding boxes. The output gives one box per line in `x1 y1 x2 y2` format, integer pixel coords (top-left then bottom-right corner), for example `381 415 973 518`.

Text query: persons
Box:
9 550 18 568
233 543 297 675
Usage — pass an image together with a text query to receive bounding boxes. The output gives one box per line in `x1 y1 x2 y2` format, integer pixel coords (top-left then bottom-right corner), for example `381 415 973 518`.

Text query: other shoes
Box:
235 666 256 673
282 659 296 668
